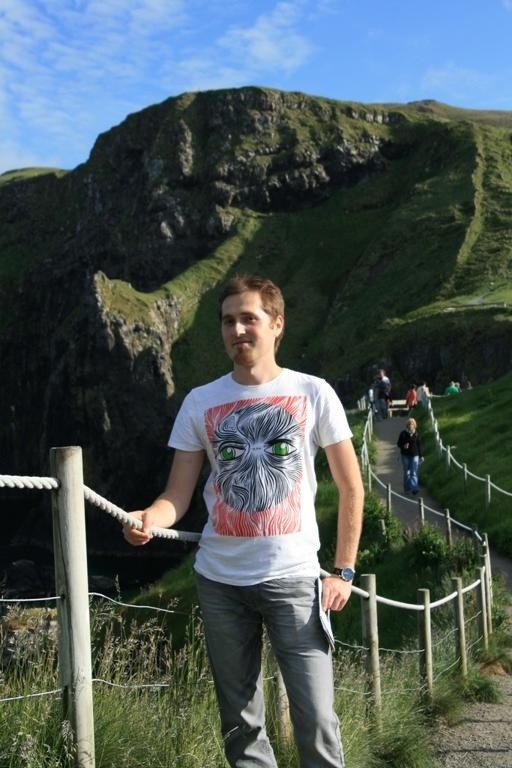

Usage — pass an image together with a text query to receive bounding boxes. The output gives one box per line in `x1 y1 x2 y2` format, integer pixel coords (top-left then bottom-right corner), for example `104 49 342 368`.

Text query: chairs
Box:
404 489 419 496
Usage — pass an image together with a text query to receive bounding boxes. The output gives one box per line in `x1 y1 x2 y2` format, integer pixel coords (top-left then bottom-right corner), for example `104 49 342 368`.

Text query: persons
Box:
120 265 372 767
370 367 473 421
396 417 425 498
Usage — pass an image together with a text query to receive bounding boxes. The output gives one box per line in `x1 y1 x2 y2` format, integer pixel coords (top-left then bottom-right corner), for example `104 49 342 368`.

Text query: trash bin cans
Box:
331 566 357 584
